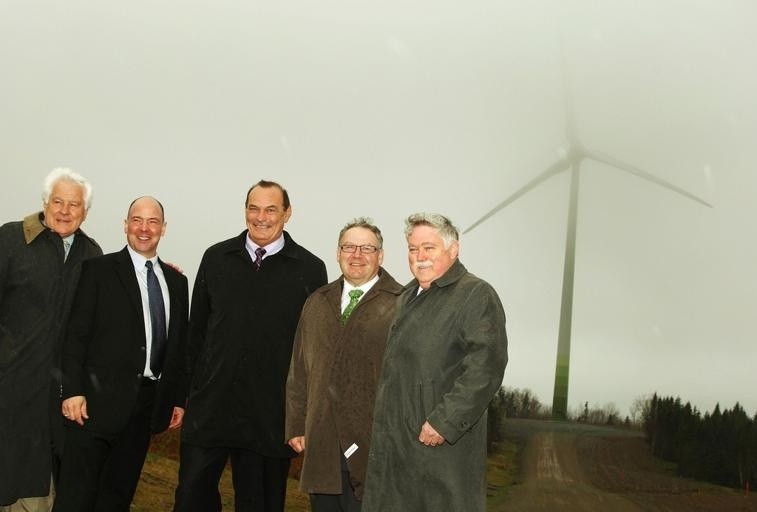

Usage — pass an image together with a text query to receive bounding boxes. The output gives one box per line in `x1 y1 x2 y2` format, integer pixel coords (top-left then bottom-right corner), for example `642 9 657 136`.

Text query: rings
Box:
64 414 69 417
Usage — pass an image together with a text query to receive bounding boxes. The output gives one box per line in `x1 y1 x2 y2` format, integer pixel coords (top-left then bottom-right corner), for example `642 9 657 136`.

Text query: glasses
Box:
338 243 381 253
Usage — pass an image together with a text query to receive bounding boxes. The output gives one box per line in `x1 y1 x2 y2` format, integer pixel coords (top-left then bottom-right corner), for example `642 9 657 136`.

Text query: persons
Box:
282 214 406 512
0 165 184 510
171 176 327 510
358 211 508 512
46 193 193 511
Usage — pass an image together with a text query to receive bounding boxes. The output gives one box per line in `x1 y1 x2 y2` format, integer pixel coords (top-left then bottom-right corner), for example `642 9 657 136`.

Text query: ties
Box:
145 260 167 380
252 246 267 274
339 288 363 326
